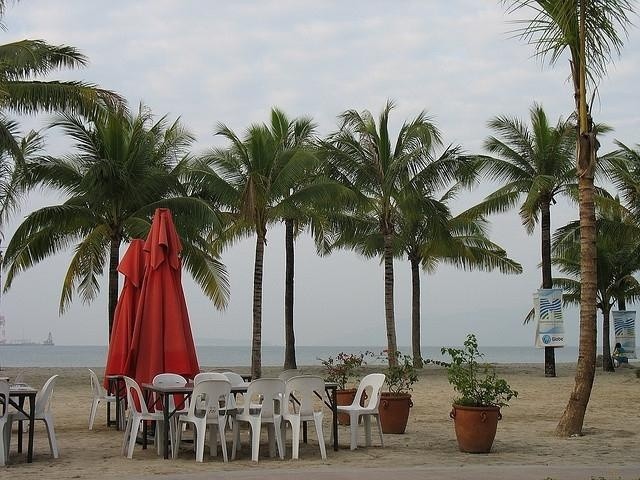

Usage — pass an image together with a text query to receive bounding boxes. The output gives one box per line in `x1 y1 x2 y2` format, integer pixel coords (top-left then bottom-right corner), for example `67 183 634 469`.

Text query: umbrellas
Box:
103 238 186 413
123 208 200 385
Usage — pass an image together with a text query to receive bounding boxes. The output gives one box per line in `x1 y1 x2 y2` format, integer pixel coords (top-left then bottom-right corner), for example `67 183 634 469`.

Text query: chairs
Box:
194 373 241 456
167 380 229 463
10 375 59 460
232 379 286 461
223 371 244 430
0 377 9 467
121 375 168 460
152 373 190 452
88 368 124 433
279 376 327 460
335 373 385 449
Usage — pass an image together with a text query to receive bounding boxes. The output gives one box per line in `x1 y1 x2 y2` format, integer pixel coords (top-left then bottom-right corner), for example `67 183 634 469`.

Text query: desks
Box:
0 384 37 463
143 382 339 460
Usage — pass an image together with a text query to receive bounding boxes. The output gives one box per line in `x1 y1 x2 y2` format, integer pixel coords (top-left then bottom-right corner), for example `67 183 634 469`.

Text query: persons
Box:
611 342 630 369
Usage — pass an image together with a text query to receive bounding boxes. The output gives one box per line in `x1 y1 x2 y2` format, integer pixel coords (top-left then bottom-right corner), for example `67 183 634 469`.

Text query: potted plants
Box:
316 352 368 425
423 334 519 453
370 350 423 434
